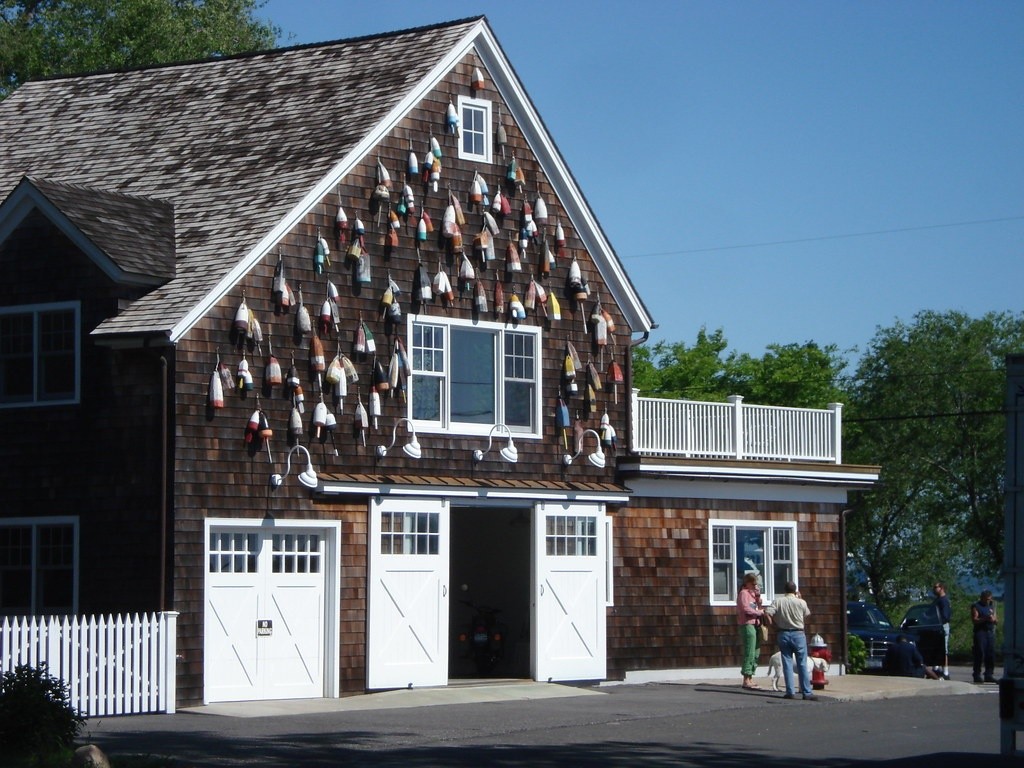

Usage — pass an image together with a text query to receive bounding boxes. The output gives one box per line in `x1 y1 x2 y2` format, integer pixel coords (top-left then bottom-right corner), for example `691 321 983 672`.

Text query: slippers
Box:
742 684 761 690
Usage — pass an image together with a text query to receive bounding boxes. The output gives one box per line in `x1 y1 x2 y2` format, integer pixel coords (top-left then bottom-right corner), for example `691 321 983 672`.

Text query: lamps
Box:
474 424 518 463
270 444 318 488
563 429 606 469
378 418 422 459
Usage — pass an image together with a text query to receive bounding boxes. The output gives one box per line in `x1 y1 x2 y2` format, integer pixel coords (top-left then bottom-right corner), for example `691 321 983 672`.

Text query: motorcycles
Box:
457 604 510 673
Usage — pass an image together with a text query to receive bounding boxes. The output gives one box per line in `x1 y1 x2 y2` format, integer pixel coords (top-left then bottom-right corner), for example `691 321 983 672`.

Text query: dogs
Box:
767 651 828 692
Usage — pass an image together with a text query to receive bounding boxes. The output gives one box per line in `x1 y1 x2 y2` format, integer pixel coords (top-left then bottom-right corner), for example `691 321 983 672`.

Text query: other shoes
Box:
974 679 983 683
984 678 996 682
936 669 950 680
803 695 818 701
784 694 795 699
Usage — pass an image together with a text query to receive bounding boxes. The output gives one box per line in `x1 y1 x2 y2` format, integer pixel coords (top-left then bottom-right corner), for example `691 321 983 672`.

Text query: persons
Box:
766 580 818 701
971 590 999 684
736 573 766 689
882 635 943 680
931 582 953 680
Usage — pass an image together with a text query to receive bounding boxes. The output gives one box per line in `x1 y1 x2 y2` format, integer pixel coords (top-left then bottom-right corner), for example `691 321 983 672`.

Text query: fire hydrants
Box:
808 632 831 689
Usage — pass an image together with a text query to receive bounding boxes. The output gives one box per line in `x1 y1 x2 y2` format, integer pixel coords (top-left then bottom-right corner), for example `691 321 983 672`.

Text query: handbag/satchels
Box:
758 616 768 641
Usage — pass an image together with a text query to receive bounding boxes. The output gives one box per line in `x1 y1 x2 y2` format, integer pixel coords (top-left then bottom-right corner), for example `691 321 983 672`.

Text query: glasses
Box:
934 586 940 588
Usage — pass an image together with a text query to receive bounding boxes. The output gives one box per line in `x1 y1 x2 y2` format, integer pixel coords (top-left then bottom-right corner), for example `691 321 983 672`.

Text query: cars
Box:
846 599 947 672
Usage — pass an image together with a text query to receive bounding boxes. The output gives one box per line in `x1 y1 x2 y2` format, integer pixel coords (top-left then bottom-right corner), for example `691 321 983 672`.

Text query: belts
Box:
780 629 803 631
